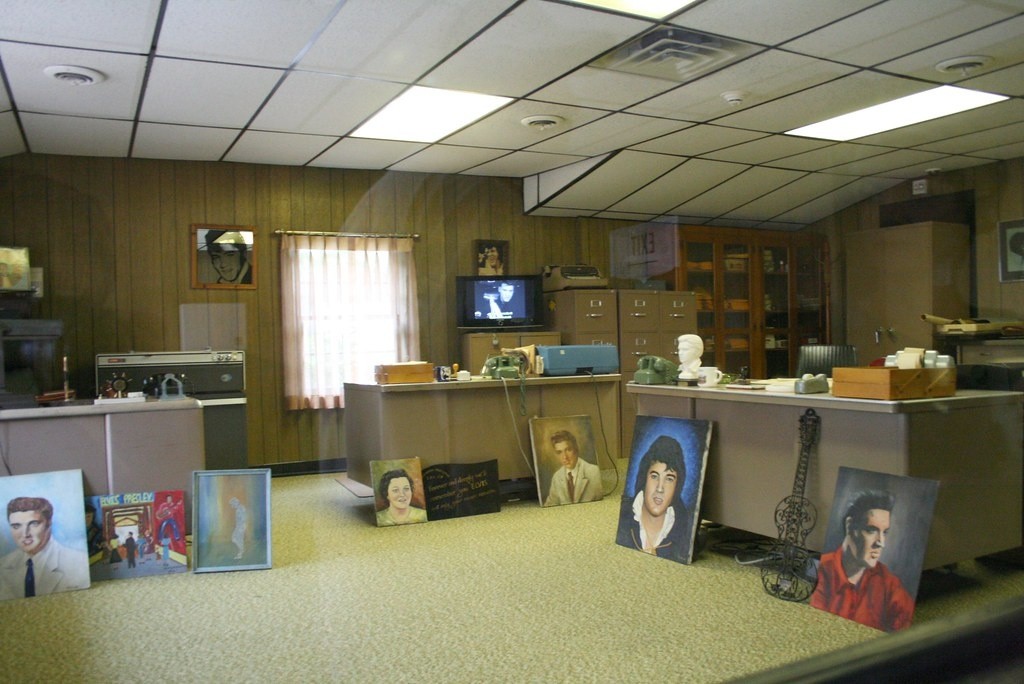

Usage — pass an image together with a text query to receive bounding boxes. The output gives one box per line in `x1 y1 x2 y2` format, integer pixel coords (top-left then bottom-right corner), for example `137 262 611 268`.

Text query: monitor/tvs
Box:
455 275 543 333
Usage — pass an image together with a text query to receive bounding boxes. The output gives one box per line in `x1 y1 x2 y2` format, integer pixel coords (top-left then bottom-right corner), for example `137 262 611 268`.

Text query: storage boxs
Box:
373 362 432 385
534 345 619 375
826 366 957 399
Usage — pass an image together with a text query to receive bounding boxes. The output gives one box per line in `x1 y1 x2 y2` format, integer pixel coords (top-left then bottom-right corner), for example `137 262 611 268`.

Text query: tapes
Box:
894 350 904 364
924 350 940 368
936 355 955 367
884 355 899 367
802 374 814 380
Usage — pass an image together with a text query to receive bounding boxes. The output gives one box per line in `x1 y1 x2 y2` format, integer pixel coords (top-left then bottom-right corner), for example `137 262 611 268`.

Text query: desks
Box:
624 377 1023 575
343 373 624 491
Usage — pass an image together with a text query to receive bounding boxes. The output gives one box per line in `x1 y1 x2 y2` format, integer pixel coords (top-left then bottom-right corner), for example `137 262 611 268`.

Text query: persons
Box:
676 334 704 379
474 281 522 318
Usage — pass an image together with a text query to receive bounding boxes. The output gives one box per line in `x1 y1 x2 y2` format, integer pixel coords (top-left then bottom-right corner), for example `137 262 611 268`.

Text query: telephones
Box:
481 355 526 380
633 354 679 385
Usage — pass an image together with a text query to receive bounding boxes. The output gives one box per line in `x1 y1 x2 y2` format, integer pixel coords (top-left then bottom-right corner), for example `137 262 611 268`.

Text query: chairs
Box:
797 343 856 380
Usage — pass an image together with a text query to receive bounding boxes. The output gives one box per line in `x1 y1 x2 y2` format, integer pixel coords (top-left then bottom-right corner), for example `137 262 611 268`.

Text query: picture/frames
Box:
998 217 1024 283
190 223 258 290
473 239 509 276
192 469 272 573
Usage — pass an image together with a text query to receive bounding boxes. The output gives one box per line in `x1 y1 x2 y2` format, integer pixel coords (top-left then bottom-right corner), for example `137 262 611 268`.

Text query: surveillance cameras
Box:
926 169 941 175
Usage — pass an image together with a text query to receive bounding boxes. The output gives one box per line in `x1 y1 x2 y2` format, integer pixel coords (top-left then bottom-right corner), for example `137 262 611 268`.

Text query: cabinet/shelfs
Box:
542 223 828 465
463 330 561 380
0 289 65 395
841 220 970 365
